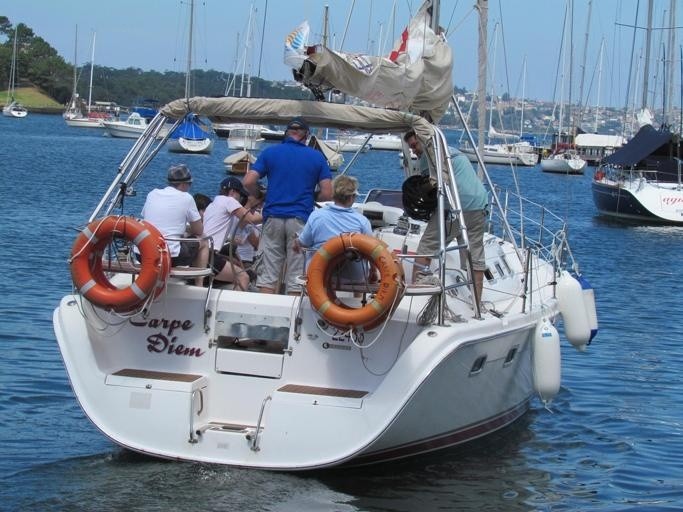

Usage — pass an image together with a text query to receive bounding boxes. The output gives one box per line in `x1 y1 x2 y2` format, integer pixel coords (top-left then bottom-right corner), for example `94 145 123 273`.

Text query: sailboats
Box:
52 0 599 474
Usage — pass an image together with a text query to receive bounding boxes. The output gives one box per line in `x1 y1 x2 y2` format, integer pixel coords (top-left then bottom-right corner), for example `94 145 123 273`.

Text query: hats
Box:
168 164 192 184
351 187 360 196
287 119 309 131
220 177 248 198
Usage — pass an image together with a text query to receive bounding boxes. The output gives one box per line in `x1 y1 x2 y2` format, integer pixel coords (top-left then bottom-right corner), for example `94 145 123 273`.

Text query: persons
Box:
242 118 333 293
235 197 258 284
292 176 378 298
403 131 489 319
134 165 210 287
192 193 212 218
202 178 264 291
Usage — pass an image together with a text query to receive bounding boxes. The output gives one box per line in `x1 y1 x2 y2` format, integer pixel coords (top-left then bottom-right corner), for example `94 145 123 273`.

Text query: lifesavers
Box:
307 234 404 332
70 216 171 312
